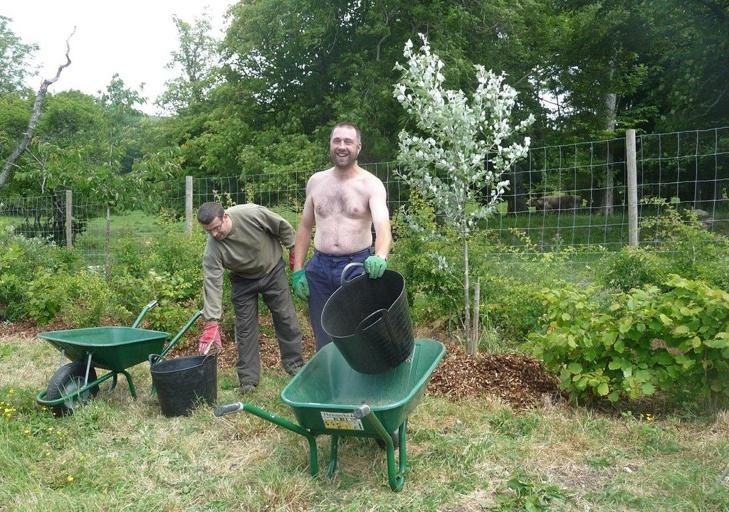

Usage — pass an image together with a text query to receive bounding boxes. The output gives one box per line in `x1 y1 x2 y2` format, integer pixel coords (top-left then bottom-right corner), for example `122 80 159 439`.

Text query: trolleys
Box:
35 300 203 417
213 337 446 492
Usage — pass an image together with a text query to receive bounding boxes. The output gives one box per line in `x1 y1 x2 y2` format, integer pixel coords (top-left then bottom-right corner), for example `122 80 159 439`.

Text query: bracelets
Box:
375 253 388 262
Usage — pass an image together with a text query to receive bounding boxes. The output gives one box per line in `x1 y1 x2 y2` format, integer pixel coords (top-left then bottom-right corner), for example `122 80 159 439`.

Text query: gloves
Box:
198 322 223 356
289 247 299 272
291 268 311 303
363 255 389 279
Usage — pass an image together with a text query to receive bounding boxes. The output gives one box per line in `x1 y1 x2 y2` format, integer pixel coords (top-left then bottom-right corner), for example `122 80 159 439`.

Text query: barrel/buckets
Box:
319 262 414 375
148 352 216 419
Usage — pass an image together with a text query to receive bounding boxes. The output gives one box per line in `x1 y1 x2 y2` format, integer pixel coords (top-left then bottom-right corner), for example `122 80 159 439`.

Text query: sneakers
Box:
283 358 309 381
238 378 258 394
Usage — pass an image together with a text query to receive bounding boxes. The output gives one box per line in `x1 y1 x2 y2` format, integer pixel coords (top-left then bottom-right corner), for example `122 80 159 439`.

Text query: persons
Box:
292 121 393 353
196 201 305 395
